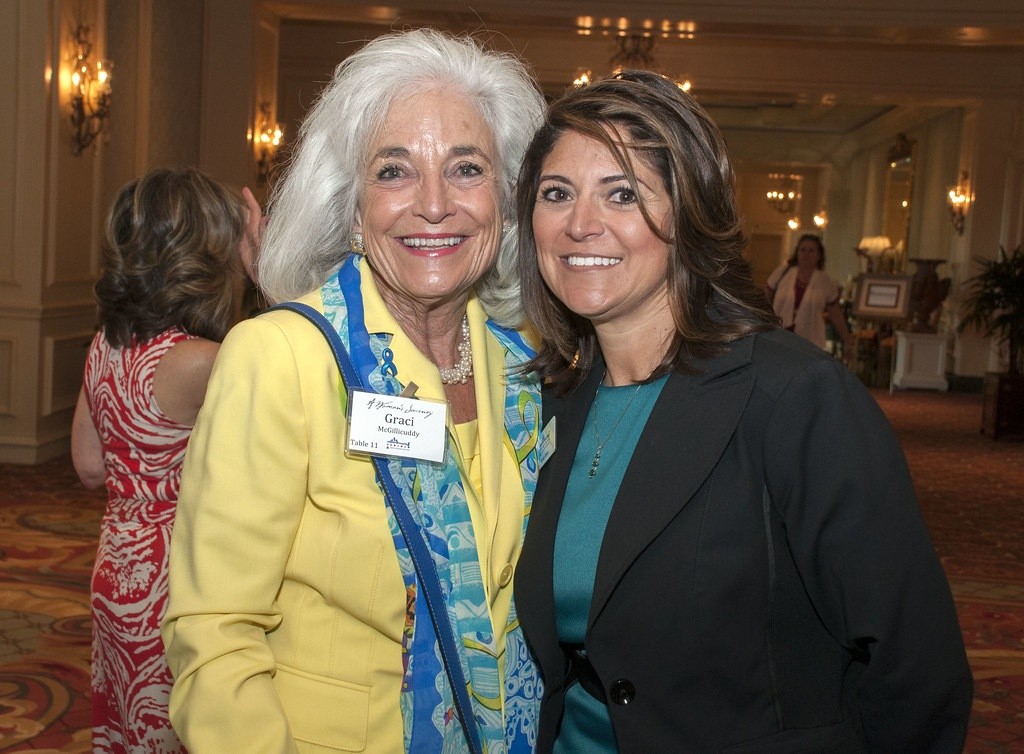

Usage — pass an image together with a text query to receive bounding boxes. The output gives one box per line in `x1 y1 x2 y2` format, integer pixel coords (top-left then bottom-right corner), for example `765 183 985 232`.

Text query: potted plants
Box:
956 243 1024 445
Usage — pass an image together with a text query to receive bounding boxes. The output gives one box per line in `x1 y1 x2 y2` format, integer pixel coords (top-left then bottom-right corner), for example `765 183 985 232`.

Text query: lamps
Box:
69 26 114 158
257 101 285 185
571 33 693 96
945 169 971 238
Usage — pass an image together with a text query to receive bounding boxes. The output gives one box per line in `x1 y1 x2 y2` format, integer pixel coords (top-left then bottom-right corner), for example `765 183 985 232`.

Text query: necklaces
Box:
438 311 473 387
589 369 654 479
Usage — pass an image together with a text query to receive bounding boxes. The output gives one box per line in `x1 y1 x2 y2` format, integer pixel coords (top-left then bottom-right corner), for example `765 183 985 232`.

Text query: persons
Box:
70 169 269 754
160 26 580 754
760 235 856 364
514 70 975 754
